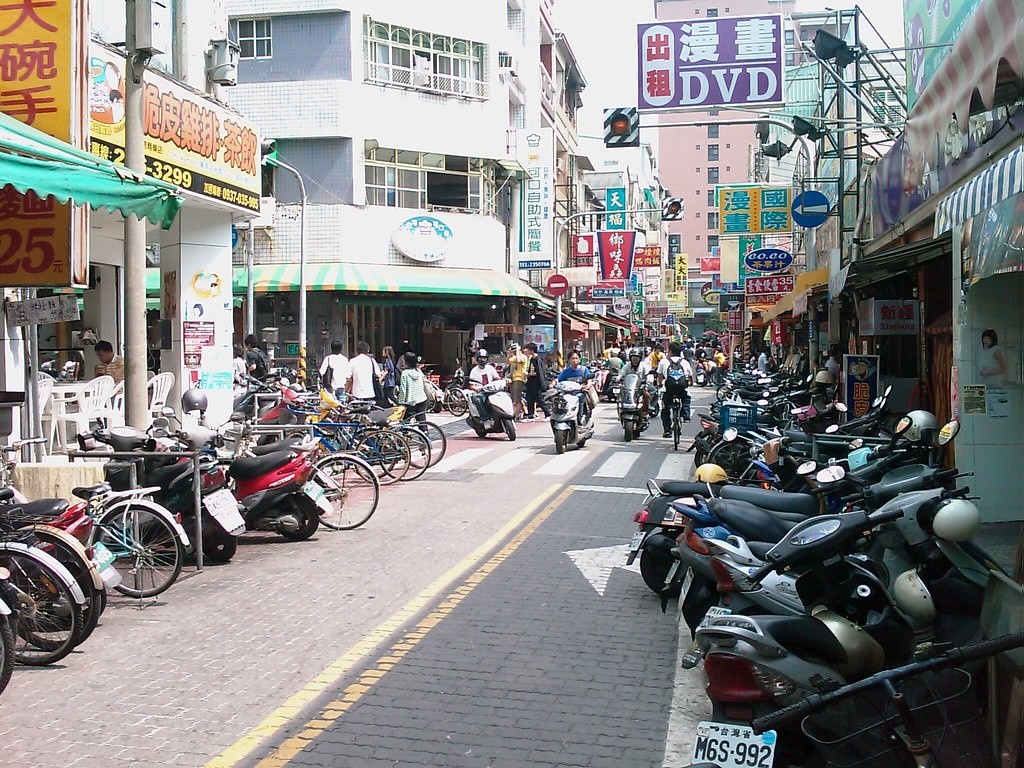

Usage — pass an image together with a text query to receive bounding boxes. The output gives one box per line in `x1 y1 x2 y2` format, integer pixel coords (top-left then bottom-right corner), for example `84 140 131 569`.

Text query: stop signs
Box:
547 275 568 296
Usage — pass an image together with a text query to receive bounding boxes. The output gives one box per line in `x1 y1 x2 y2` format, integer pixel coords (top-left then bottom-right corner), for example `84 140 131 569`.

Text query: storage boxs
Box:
719 404 757 434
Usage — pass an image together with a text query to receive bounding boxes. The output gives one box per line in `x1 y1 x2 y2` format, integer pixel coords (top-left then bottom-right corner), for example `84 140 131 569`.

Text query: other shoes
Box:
418 443 432 451
520 416 552 423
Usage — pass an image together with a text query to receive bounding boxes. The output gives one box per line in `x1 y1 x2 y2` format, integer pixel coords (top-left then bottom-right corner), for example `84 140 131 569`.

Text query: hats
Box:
62 360 77 371
506 343 521 353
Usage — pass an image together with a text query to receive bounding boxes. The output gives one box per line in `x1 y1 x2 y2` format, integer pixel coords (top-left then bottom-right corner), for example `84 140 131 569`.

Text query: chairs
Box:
37 371 176 455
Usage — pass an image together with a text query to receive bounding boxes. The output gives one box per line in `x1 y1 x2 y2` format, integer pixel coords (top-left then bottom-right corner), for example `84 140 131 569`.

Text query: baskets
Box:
801 666 993 768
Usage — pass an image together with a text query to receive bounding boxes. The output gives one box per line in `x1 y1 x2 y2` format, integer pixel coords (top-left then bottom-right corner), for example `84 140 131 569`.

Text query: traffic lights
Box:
812 28 855 68
662 196 684 218
604 108 638 143
760 115 822 161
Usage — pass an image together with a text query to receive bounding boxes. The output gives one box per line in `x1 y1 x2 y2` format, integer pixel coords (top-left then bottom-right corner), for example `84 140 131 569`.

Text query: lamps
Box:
791 116 904 142
812 29 954 68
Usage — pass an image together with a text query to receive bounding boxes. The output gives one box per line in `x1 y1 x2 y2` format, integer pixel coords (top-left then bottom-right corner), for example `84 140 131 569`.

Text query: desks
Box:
51 381 115 453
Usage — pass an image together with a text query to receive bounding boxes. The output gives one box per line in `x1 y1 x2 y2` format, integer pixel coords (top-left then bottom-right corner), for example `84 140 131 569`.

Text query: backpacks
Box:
717 352 729 370
391 358 402 385
665 357 689 394
417 368 445 404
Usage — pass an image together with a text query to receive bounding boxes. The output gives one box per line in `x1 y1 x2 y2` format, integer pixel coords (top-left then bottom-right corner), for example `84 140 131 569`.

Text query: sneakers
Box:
484 420 491 430
683 417 691 423
92 429 106 442
663 427 670 437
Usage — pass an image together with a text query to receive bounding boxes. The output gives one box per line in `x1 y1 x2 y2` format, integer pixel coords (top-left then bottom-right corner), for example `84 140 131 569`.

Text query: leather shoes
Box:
642 406 655 412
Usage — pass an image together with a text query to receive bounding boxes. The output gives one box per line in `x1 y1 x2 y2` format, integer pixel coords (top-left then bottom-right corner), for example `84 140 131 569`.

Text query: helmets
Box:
904 410 939 443
894 568 936 620
815 370 835 388
695 463 728 483
159 438 180 465
629 348 643 360
181 389 207 414
931 490 981 542
475 349 489 361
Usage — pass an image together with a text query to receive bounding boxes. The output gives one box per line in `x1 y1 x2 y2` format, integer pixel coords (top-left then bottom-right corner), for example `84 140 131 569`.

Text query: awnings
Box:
52 262 543 310
764 266 830 324
827 230 952 304
1 110 185 231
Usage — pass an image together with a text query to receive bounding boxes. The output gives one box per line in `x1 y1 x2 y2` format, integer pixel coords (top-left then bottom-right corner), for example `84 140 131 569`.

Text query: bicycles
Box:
659 384 692 451
0 488 122 697
0 437 194 610
240 356 472 486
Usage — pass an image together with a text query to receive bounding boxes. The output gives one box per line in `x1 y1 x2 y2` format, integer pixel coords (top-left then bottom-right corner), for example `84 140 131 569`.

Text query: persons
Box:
379 345 399 408
232 335 267 398
399 351 428 456
978 329 1007 388
548 349 592 426
346 341 382 412
318 340 352 406
505 342 551 423
615 348 655 411
468 348 512 431
656 342 693 437
748 346 773 374
94 340 124 395
597 339 726 393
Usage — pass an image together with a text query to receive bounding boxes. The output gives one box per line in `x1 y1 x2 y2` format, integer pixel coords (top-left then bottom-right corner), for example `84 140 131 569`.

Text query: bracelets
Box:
552 379 558 386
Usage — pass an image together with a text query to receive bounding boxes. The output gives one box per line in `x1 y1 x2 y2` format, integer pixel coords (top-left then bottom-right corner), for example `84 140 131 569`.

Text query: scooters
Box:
611 366 656 441
709 366 843 425
544 367 595 454
625 419 1024 768
78 388 380 565
696 358 709 387
604 374 620 402
712 363 784 401
685 385 940 492
466 364 517 441
517 360 609 411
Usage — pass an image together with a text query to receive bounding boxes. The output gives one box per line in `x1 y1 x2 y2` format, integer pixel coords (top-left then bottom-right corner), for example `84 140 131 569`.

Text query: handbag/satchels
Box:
323 356 334 389
582 366 599 409
370 357 385 400
246 349 270 376
